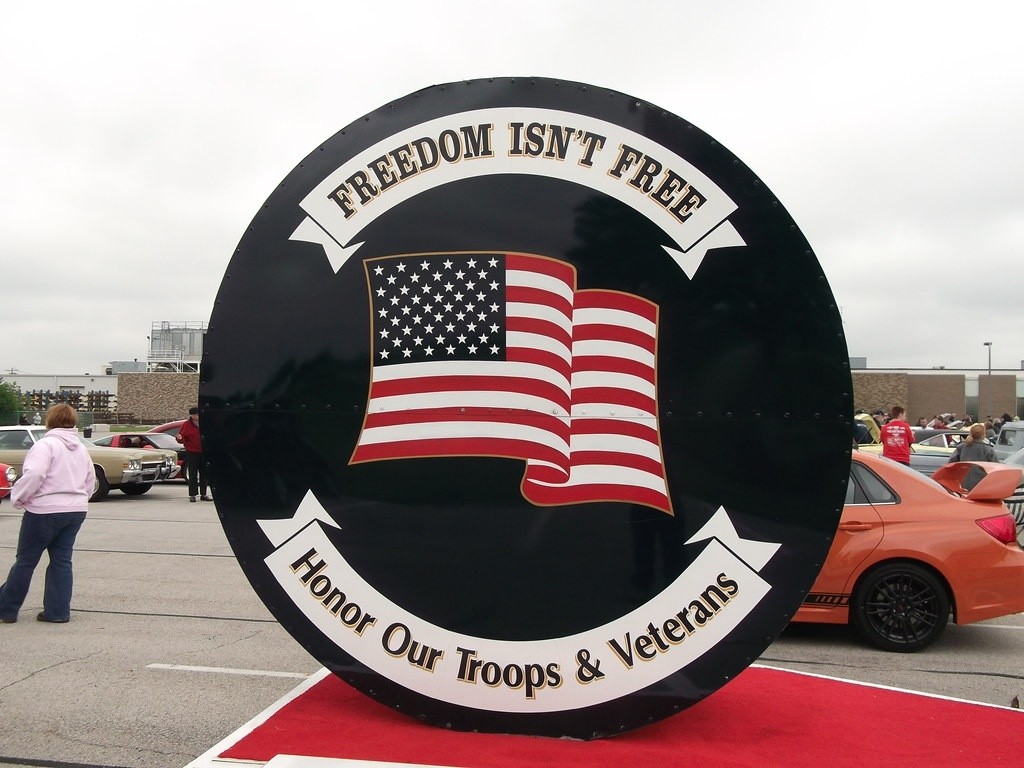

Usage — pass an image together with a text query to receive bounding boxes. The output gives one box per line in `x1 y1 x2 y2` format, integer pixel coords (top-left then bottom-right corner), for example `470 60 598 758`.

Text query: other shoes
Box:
37 611 69 624
190 496 196 503
201 495 214 501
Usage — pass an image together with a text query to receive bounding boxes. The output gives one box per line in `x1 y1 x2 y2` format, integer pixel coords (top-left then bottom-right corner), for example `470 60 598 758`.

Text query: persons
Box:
175 408 214 502
0 403 95 623
855 406 1024 492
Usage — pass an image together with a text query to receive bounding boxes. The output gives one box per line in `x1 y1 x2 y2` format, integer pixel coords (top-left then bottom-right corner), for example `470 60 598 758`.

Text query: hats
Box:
874 411 884 415
855 409 864 415
189 407 198 414
934 415 943 420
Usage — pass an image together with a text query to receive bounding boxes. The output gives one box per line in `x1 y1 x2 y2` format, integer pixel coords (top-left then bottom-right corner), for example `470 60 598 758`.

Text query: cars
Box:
0 423 182 502
132 418 189 444
851 413 1024 491
90 431 209 485
0 461 18 506
788 446 1024 654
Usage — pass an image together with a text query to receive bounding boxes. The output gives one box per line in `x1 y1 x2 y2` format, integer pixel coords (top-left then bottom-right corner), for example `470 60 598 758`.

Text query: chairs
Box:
121 437 130 447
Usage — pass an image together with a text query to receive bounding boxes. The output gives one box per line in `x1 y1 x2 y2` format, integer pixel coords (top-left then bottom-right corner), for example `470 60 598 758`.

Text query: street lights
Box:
982 341 993 418
